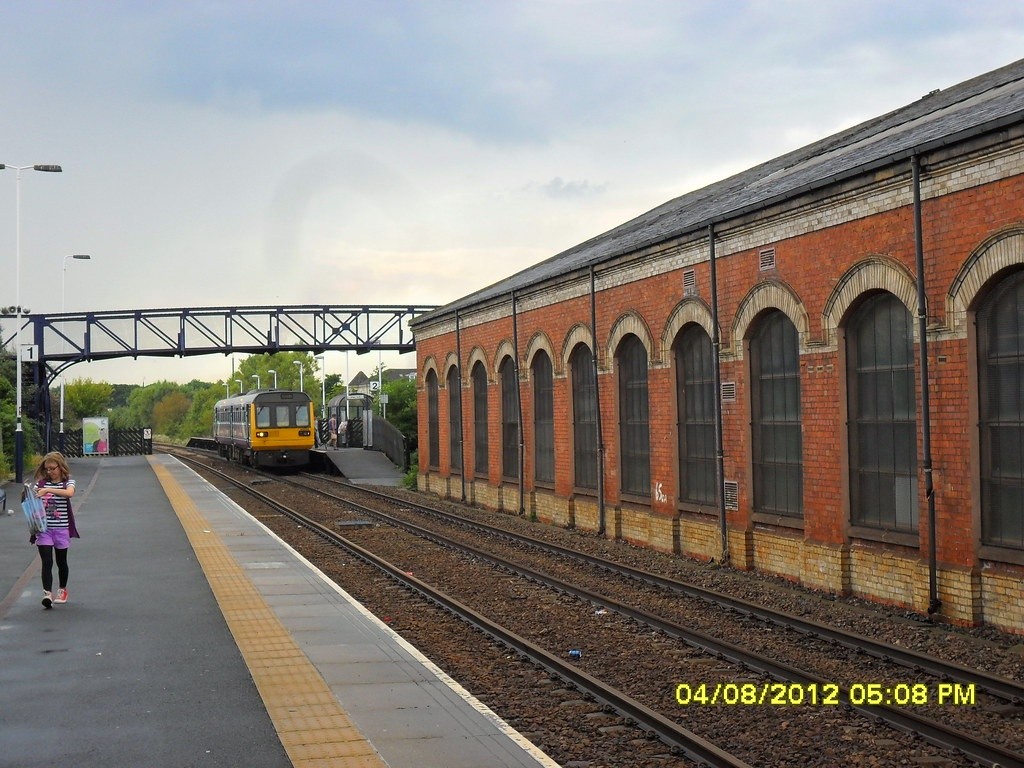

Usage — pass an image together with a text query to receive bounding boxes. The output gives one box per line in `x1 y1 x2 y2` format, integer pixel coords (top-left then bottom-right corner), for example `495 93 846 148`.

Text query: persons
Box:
22 451 80 609
314 415 323 449
325 413 339 451
93 426 107 452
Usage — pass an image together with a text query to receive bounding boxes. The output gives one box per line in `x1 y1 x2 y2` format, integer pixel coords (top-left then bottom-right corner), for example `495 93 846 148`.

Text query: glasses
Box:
44 465 59 472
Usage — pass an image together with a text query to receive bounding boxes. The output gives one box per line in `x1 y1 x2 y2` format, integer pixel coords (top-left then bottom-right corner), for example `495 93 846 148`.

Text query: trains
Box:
213 387 316 473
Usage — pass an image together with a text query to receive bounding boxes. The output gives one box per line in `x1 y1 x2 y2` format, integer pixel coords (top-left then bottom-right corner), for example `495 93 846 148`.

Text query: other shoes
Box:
316 447 321 449
334 448 339 450
319 443 323 446
325 444 328 450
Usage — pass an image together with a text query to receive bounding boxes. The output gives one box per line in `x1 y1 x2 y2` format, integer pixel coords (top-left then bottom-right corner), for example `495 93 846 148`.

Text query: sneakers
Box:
41 589 53 609
54 587 68 603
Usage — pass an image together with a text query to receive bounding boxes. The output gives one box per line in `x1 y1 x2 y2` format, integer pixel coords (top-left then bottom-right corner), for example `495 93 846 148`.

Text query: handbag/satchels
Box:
21 483 47 534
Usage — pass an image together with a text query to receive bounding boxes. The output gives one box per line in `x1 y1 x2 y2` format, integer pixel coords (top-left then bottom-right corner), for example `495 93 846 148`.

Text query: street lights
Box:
267 370 277 389
293 360 304 392
315 355 327 420
222 383 230 399
252 375 260 390
373 339 382 417
57 252 92 458
0 161 63 486
339 349 349 420
235 380 243 394
